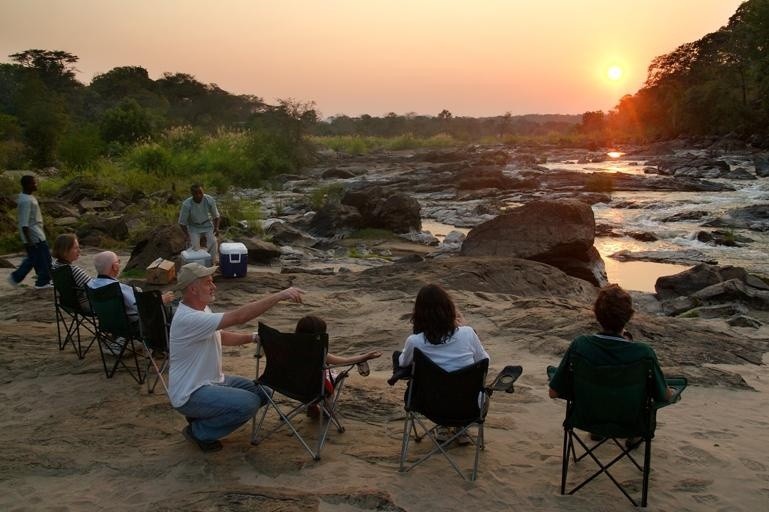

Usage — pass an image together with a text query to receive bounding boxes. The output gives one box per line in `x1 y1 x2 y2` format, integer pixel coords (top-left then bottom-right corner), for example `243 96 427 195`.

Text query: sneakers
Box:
4 273 19 290
182 424 223 453
33 282 51 290
307 405 320 418
434 424 449 441
455 427 474 446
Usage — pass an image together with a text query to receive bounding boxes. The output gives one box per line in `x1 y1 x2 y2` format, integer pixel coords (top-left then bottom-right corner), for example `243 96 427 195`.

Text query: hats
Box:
172 262 219 294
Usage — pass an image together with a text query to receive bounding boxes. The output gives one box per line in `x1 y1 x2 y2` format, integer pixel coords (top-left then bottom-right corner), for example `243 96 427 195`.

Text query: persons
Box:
178 182 221 264
88 249 176 358
295 313 384 420
52 232 92 313
549 282 673 449
399 282 490 445
8 175 54 291
168 262 308 455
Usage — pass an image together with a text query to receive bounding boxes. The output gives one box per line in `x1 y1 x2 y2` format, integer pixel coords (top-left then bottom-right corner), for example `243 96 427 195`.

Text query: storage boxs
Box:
181 250 212 267
146 257 175 285
219 243 248 278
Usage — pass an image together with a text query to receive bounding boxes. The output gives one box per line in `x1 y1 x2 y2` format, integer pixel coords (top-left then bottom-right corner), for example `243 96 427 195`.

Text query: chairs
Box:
388 347 522 481
84 282 168 384
132 284 174 394
250 320 369 462
547 360 688 507
48 265 100 360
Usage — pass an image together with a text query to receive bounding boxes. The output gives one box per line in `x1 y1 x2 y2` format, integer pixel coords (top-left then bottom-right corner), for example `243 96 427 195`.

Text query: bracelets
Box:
252 331 259 343
